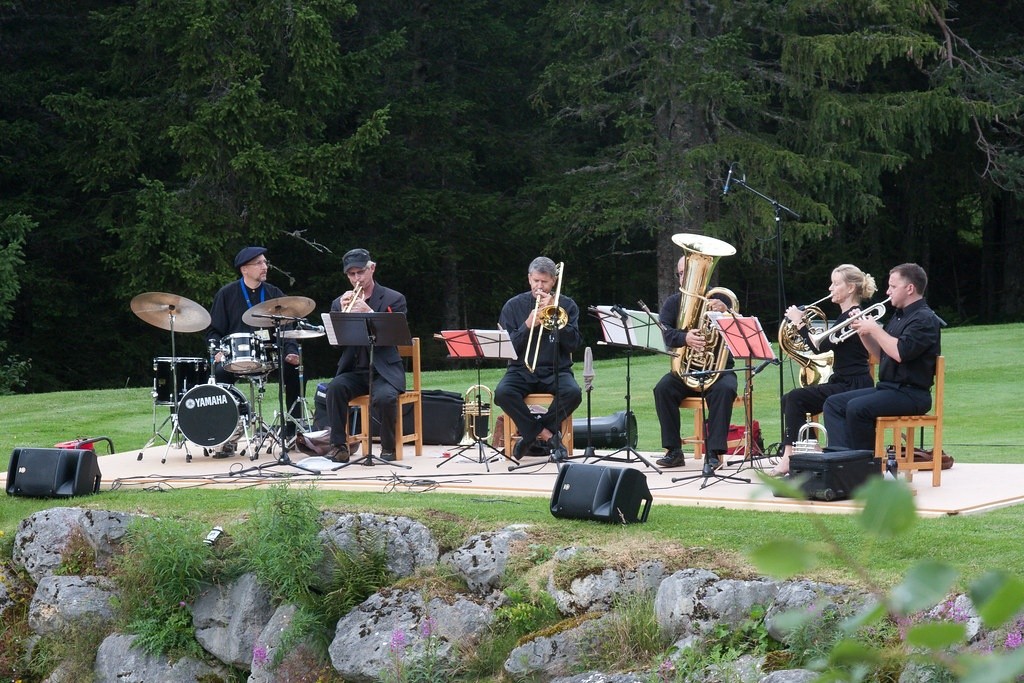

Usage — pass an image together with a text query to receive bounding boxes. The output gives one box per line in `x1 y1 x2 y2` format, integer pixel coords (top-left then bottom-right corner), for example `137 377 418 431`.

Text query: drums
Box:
154 356 208 407
176 382 252 449
221 332 265 373
233 344 280 379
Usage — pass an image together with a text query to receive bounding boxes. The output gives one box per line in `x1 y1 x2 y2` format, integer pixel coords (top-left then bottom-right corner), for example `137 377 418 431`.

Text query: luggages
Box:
788 450 874 502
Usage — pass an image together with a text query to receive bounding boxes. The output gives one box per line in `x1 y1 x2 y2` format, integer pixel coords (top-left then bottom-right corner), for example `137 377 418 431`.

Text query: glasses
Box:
243 260 270 267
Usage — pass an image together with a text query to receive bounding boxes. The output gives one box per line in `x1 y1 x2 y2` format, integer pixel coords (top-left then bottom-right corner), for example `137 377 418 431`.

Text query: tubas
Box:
669 232 740 394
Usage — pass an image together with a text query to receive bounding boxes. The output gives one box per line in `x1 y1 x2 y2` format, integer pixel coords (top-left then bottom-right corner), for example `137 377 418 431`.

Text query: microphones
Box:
305 323 325 333
724 166 734 195
748 359 770 380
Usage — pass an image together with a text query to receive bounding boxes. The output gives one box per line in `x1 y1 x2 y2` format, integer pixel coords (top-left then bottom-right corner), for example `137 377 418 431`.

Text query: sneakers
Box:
656 446 686 466
381 445 396 461
324 444 350 463
704 450 722 469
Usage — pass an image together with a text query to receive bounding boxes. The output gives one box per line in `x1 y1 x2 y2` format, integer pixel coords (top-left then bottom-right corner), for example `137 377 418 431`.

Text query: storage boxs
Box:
678 397 752 459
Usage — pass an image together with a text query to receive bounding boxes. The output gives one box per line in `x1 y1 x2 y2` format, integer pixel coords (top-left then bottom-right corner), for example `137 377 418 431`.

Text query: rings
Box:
356 307 358 309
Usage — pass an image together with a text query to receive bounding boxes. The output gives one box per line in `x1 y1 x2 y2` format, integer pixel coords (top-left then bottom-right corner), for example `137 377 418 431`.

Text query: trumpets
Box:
807 296 893 352
341 280 366 313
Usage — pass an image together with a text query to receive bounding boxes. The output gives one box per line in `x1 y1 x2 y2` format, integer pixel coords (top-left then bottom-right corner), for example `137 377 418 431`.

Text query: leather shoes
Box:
513 434 538 460
546 429 567 459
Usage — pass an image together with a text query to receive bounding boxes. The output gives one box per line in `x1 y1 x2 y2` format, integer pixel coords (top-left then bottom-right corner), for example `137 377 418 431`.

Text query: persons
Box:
768 263 876 477
652 254 738 471
324 249 408 463
823 263 942 470
204 247 308 452
493 255 583 472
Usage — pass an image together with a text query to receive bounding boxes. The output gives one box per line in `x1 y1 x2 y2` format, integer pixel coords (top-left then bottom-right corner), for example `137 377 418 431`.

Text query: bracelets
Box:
795 321 803 327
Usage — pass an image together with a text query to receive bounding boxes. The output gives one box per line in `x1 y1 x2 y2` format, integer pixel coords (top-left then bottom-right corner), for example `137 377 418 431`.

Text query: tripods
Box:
436 300 782 487
227 308 414 475
137 308 209 464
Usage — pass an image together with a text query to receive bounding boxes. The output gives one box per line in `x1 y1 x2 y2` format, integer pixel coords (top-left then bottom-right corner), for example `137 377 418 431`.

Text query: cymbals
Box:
273 330 327 339
130 290 212 335
241 295 317 328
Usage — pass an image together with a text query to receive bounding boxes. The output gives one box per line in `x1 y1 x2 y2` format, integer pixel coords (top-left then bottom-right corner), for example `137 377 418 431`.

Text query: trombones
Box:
523 261 569 374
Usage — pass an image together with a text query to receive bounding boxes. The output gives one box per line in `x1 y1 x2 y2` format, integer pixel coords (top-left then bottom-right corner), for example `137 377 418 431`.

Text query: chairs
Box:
344 337 423 461
806 355 945 487
504 394 573 460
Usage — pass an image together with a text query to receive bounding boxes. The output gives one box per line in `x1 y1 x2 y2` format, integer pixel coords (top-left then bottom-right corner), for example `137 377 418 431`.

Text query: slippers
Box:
767 468 790 478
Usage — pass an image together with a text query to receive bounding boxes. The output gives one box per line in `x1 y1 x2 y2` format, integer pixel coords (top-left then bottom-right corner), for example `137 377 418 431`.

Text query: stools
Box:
231 372 270 447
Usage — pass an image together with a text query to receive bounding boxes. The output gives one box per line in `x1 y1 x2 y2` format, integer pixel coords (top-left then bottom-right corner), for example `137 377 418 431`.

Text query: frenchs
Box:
778 293 833 389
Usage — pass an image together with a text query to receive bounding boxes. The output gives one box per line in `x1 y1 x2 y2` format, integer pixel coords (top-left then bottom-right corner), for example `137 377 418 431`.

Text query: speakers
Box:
550 464 653 524
6 447 101 498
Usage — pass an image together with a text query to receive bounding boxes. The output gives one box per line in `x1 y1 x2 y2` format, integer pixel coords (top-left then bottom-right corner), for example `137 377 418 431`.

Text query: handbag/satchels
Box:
701 420 764 456
899 447 954 471
401 389 466 445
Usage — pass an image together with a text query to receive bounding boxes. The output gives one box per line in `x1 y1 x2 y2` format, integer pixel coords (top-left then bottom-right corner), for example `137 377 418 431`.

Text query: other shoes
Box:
286 435 296 450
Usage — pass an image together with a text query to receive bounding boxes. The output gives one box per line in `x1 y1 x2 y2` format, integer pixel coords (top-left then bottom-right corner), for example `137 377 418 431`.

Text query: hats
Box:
234 246 268 268
342 249 371 275
